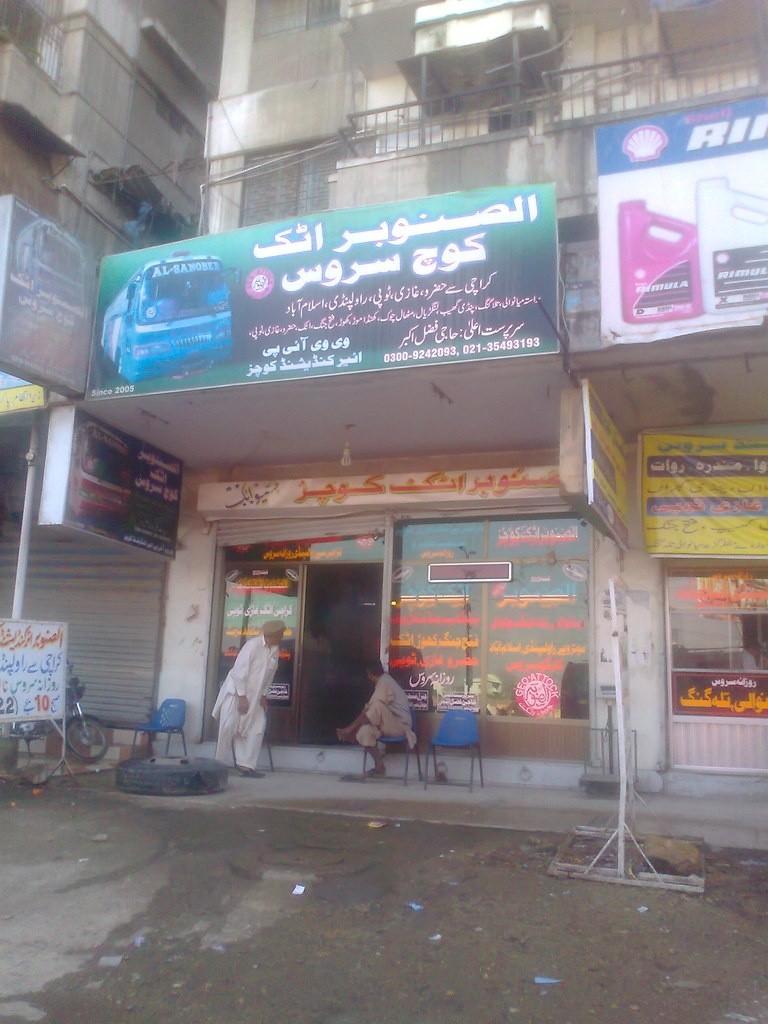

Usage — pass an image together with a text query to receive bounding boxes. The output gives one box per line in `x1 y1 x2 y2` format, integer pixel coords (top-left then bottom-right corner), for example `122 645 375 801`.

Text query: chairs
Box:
424 710 483 792
131 699 188 757
362 710 422 785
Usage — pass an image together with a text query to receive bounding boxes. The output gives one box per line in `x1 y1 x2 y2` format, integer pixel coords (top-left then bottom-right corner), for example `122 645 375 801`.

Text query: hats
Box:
262 620 285 637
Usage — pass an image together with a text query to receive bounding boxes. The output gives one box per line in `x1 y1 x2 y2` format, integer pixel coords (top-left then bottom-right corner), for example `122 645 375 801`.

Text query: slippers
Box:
364 769 387 778
338 774 364 784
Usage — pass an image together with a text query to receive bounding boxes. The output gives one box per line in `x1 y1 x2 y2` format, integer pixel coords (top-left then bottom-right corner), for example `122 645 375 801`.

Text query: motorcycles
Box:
0 659 109 763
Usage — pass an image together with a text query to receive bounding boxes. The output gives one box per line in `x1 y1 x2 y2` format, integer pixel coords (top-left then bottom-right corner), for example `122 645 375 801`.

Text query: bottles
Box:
618 175 768 323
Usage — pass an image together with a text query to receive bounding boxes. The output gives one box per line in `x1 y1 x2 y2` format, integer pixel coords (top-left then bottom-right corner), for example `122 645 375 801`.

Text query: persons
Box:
298 619 332 730
211 621 286 778
336 656 412 780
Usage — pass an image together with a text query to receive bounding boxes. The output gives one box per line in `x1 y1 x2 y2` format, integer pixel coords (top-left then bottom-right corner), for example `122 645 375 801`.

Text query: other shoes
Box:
237 770 265 778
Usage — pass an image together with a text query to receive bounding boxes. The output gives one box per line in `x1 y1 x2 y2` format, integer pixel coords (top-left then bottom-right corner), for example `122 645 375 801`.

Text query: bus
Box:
99 252 233 384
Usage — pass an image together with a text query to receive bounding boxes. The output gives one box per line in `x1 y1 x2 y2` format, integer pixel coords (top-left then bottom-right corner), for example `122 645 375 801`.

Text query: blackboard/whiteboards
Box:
0 619 81 783
